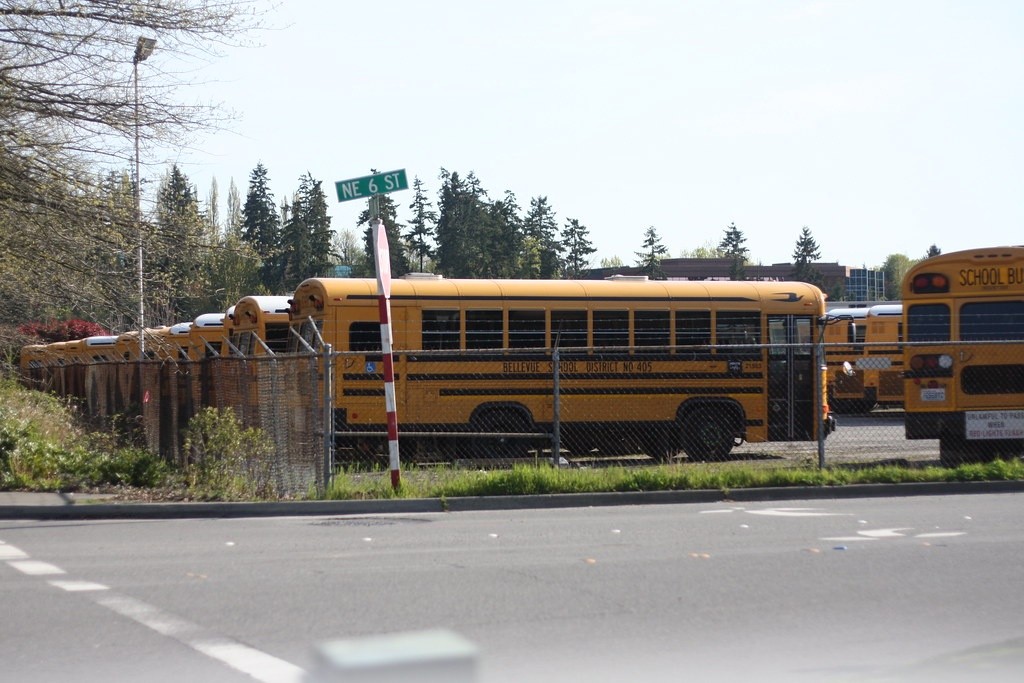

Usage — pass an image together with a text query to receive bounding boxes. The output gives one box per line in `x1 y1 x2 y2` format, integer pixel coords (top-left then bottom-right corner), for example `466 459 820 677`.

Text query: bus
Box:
21 278 857 460
823 246 1023 468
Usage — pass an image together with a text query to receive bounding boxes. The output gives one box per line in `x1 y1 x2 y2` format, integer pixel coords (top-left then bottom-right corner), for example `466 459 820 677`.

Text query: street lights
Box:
132 34 157 356
